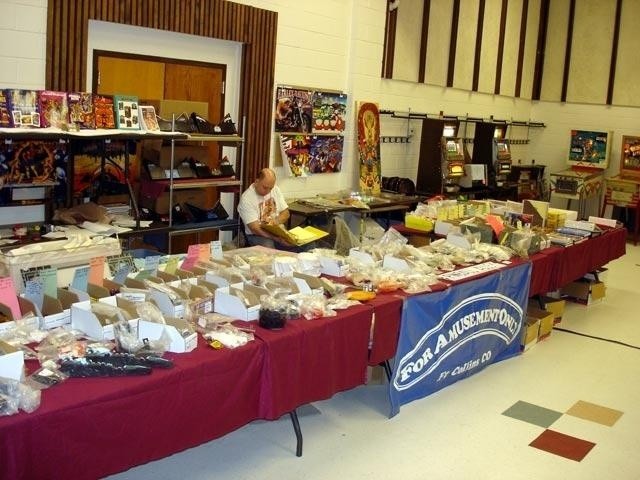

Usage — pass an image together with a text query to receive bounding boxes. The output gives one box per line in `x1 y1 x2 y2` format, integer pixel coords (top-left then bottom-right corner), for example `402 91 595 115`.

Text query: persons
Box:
238 168 316 254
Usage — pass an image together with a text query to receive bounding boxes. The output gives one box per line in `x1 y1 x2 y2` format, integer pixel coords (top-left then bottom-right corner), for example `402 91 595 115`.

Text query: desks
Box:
2 224 627 480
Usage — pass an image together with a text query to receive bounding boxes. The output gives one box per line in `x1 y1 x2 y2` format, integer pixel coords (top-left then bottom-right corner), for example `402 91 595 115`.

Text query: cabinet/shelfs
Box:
147 112 247 256
